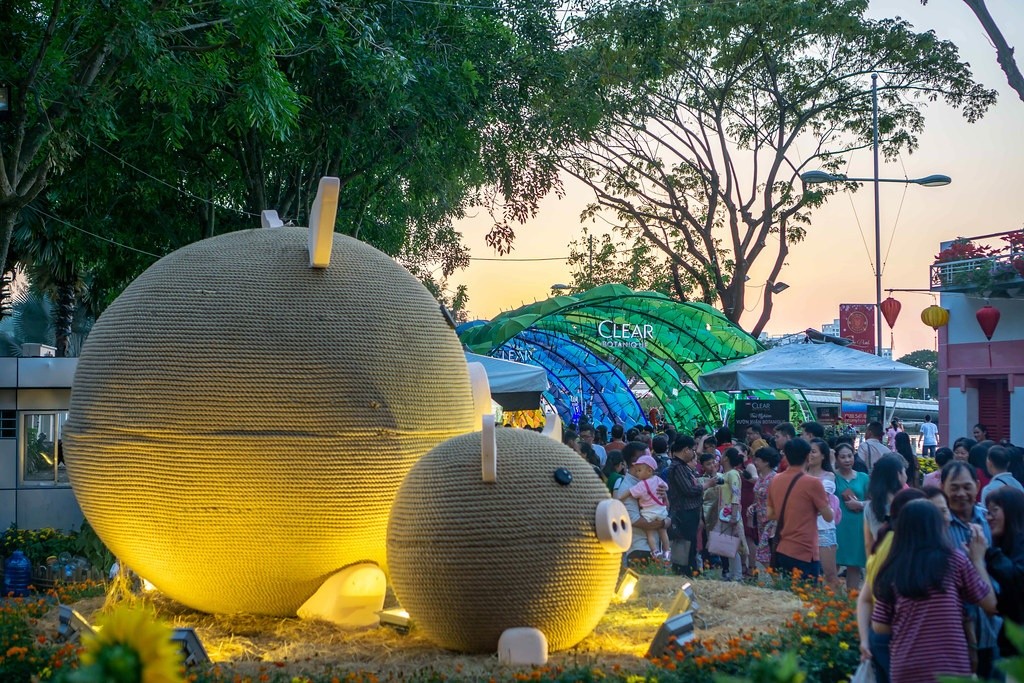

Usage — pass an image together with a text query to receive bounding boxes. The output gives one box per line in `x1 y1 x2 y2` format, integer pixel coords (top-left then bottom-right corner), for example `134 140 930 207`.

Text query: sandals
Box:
742 563 758 581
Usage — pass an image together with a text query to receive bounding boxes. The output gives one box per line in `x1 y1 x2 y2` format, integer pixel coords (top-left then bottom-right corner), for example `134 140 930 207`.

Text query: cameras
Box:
715 478 724 485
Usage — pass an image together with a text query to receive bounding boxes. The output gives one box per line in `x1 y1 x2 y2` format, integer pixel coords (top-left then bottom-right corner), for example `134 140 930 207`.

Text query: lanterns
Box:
921 304 949 350
976 306 1000 368
880 297 901 350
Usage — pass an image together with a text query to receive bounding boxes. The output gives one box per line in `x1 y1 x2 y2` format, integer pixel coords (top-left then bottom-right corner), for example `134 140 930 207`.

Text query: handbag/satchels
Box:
747 508 758 529
852 661 877 683
719 505 740 522
706 529 740 558
770 536 783 569
843 489 861 512
667 538 692 565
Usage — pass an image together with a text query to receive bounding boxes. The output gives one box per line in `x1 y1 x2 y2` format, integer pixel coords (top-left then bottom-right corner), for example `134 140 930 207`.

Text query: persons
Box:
562 416 1024 683
496 422 543 433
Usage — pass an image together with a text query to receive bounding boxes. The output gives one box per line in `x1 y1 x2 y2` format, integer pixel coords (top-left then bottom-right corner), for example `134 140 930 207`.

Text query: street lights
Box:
799 170 951 405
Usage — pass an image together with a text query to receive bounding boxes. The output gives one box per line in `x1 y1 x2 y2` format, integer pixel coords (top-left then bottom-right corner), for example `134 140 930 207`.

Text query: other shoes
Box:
663 551 670 563
651 550 661 560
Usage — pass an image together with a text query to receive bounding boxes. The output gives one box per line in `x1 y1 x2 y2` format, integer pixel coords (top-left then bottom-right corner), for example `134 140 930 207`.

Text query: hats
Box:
632 456 657 471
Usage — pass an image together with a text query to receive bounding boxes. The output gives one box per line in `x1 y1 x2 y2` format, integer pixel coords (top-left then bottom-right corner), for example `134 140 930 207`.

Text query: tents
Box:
464 350 550 412
698 339 929 437
